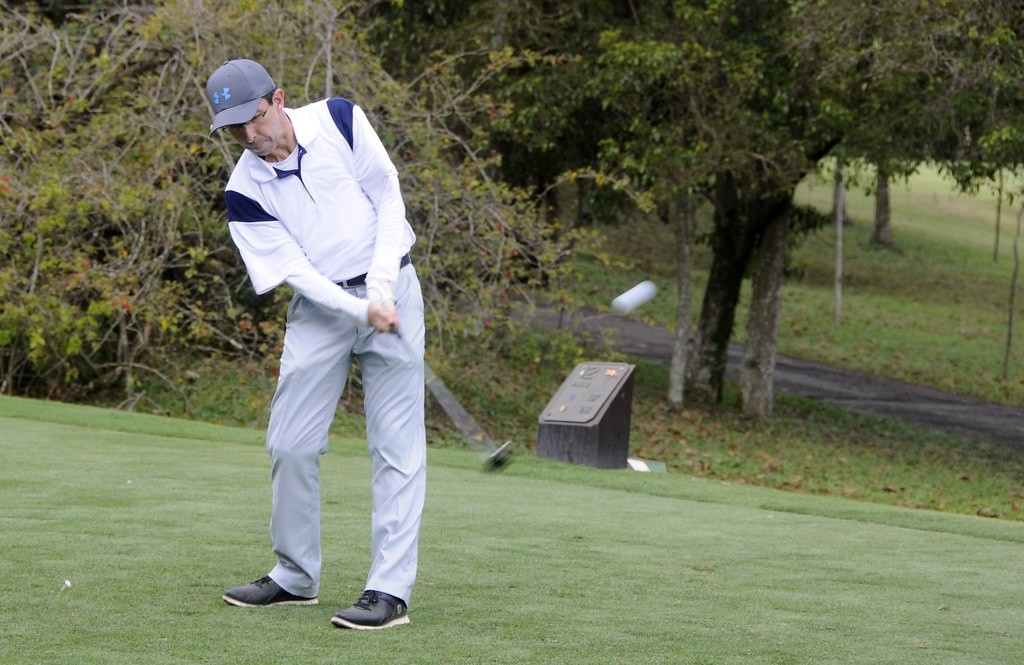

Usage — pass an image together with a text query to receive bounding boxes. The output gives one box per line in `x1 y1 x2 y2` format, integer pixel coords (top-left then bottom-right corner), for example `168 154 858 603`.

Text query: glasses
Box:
222 93 273 135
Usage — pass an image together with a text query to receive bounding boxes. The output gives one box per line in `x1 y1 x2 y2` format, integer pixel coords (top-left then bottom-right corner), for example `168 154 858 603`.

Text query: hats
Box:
206 58 276 139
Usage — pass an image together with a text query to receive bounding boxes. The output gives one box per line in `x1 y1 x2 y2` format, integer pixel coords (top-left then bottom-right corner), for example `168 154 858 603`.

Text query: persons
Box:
206 57 423 629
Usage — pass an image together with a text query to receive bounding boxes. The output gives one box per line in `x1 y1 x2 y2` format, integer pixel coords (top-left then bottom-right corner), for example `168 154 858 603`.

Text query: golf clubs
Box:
388 317 516 474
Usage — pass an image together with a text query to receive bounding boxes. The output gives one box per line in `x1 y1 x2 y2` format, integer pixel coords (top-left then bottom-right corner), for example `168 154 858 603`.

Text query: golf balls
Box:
610 278 657 318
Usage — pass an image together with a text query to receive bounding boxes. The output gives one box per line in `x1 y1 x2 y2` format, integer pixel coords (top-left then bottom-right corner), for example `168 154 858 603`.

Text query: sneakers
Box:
330 590 411 630
222 575 319 608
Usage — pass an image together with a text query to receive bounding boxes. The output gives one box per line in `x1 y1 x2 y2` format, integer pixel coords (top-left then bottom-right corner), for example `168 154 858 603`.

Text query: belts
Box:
336 253 411 289
